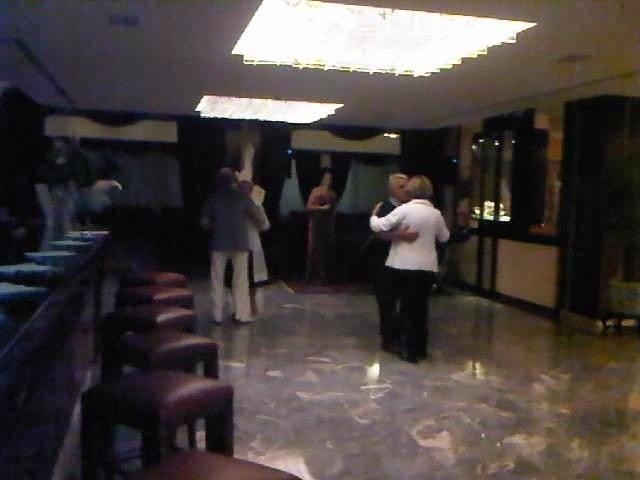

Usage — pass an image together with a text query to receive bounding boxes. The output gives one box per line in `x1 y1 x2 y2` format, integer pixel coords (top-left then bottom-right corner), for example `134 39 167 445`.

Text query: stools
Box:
83 369 234 480
100 307 198 371
112 330 219 384
135 447 300 480
115 287 195 310
118 271 185 288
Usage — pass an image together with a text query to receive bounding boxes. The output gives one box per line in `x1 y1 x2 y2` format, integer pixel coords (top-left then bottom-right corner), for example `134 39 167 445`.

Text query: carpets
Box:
275 269 379 296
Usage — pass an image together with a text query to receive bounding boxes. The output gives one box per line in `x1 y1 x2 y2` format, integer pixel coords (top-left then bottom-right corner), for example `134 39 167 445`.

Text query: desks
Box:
0 223 114 479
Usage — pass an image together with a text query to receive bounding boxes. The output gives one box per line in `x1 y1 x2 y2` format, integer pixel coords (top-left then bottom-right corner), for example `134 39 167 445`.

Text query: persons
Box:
201 180 272 317
304 172 337 286
201 168 266 324
365 174 418 354
368 174 450 362
